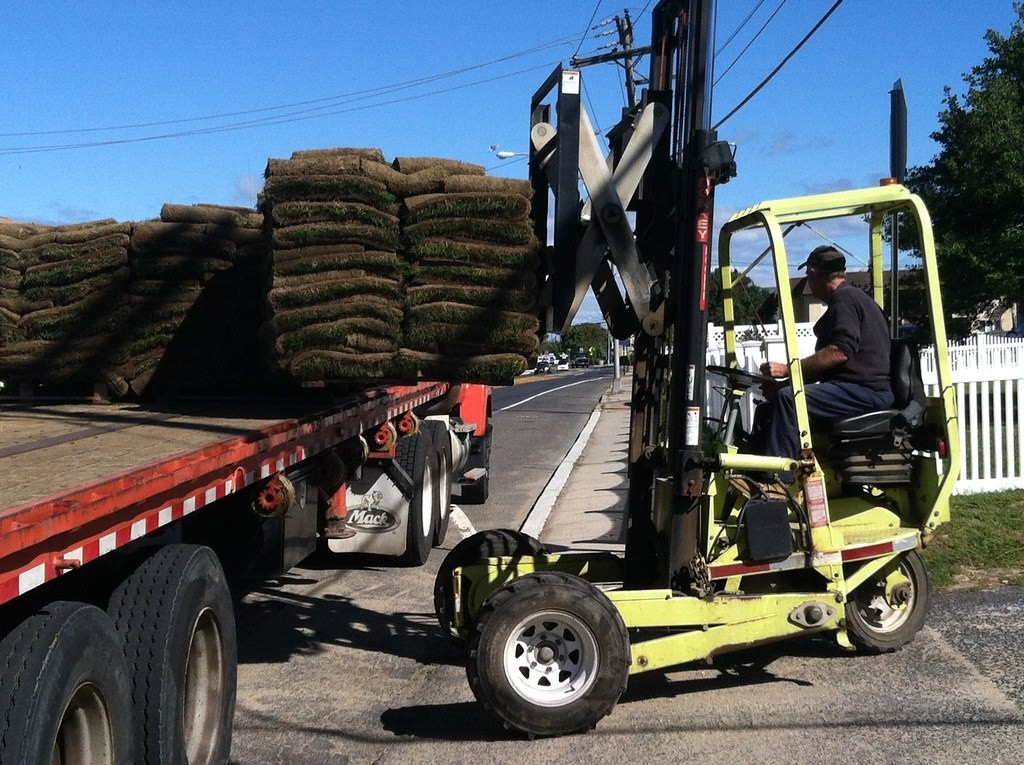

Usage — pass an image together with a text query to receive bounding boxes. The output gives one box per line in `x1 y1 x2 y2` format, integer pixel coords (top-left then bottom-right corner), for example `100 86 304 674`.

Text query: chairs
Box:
827 336 928 438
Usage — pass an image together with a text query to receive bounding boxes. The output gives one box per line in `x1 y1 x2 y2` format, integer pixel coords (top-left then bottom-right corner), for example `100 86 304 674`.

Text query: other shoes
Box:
729 477 785 499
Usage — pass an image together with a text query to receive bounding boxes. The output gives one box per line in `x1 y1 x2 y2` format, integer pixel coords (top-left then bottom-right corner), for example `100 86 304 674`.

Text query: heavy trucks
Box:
0 377 497 765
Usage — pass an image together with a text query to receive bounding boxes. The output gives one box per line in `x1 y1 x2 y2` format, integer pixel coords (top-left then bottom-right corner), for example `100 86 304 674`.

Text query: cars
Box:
534 350 590 374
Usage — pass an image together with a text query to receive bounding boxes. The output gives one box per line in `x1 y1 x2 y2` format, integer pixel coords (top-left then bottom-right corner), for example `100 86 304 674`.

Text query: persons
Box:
730 244 902 504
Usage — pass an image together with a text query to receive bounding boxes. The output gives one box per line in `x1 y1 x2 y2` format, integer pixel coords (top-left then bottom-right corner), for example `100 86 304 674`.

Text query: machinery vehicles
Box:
431 2 962 741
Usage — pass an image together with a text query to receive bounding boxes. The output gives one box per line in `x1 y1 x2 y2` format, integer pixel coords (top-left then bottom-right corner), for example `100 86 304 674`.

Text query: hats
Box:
798 246 844 273
